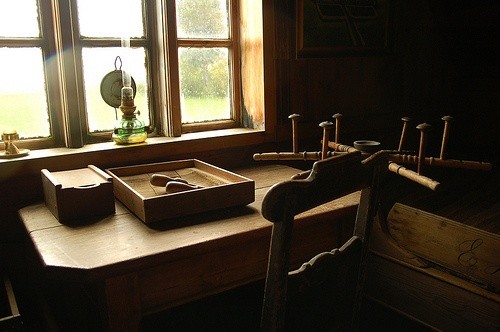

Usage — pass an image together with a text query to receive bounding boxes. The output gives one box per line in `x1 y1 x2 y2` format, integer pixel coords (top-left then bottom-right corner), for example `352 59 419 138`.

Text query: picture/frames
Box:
101 70 136 107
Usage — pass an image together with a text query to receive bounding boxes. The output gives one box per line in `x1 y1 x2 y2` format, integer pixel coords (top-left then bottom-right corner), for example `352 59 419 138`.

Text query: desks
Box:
17 164 360 332
